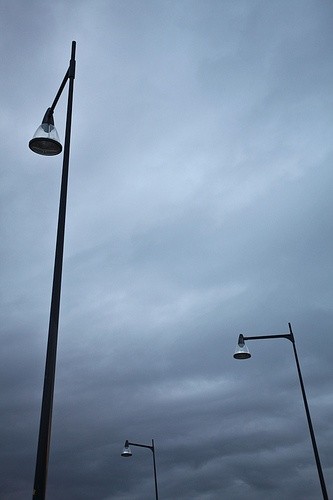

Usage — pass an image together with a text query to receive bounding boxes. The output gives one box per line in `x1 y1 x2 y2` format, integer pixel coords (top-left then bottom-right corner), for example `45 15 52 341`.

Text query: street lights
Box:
28 41 77 499
233 321 330 499
121 438 158 500
